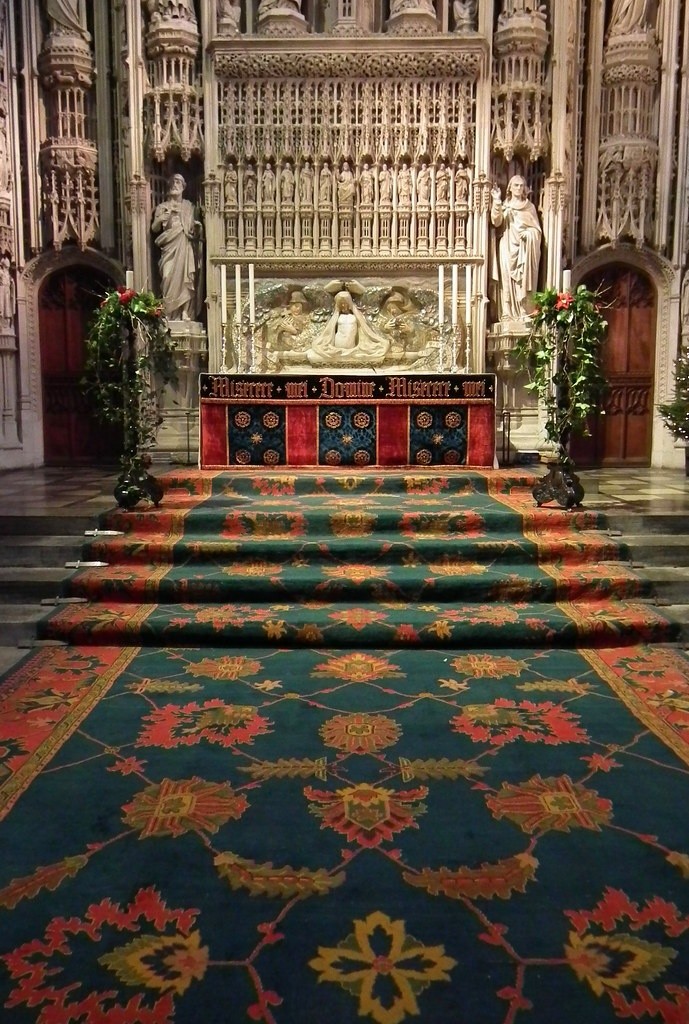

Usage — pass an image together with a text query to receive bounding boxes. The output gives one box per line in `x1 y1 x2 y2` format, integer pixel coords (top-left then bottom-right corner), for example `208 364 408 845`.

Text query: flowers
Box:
517 277 613 467
84 285 172 471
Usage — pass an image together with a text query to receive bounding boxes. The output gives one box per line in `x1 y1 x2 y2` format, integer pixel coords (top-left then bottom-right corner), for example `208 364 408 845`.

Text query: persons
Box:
490 174 543 322
42 0 653 369
